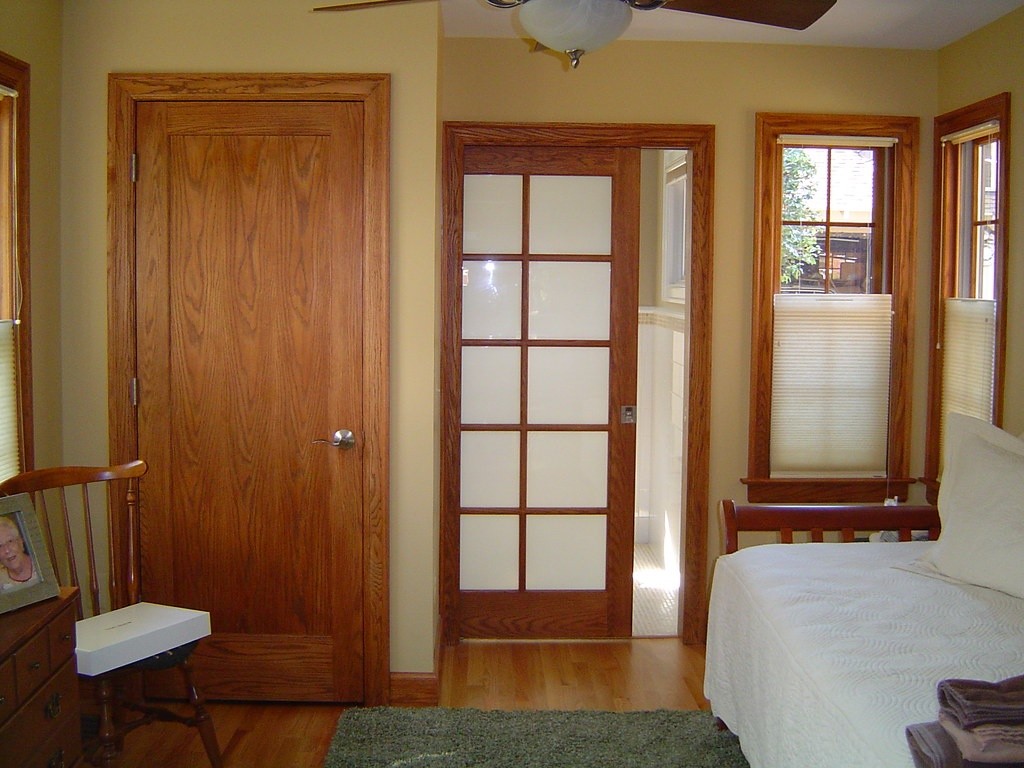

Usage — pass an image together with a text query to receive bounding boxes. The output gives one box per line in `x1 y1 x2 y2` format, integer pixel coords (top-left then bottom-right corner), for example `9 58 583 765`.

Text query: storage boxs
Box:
73 601 212 677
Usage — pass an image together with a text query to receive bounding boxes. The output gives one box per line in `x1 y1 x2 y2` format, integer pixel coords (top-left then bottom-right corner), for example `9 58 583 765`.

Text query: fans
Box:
312 0 840 31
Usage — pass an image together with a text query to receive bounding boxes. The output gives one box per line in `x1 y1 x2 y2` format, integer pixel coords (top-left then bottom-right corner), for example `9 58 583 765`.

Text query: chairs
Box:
0 460 224 768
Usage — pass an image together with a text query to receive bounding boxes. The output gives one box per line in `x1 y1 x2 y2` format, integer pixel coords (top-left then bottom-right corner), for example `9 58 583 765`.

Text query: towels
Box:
905 674 1024 768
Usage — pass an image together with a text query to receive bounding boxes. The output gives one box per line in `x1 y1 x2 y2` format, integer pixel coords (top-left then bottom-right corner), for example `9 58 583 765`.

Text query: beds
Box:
702 493 1024 768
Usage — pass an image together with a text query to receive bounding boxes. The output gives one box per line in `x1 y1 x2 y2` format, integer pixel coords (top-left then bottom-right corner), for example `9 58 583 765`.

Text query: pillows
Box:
889 411 1024 600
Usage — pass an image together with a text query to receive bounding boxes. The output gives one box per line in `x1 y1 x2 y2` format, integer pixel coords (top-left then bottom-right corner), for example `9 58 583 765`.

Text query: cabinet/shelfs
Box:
0 586 85 768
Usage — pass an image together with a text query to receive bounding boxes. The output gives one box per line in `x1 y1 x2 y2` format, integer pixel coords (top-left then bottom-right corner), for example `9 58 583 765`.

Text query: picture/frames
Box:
0 492 61 615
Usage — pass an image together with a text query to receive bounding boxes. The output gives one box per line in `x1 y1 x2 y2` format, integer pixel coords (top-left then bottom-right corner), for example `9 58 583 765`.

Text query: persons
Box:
0 516 39 594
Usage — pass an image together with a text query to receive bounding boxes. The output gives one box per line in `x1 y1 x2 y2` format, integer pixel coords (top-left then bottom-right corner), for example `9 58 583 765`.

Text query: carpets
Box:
321 705 752 768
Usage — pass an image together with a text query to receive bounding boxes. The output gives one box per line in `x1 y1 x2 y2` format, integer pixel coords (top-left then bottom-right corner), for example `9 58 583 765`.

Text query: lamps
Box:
517 0 634 69
